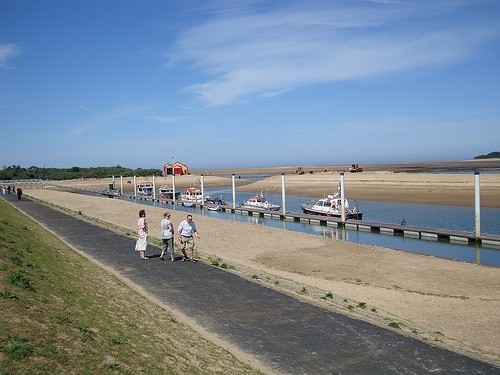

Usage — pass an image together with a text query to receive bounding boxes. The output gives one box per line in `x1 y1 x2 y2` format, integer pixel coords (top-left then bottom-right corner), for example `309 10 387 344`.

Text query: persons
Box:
159 212 176 262
178 215 201 263
2 183 22 200
134 209 149 260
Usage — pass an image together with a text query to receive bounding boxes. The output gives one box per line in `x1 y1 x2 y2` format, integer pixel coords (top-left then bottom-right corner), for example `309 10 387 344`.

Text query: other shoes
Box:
160 256 164 261
140 256 149 260
171 258 176 262
190 259 196 263
182 255 187 262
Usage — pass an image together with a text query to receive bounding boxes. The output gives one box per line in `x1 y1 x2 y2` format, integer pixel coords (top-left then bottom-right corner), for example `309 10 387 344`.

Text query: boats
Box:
301 182 362 220
240 189 281 212
136 178 230 213
101 184 120 196
349 163 364 172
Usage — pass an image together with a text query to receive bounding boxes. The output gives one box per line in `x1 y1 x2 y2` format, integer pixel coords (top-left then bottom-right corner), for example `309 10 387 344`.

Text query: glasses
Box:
166 215 171 217
143 213 146 215
188 218 192 220
